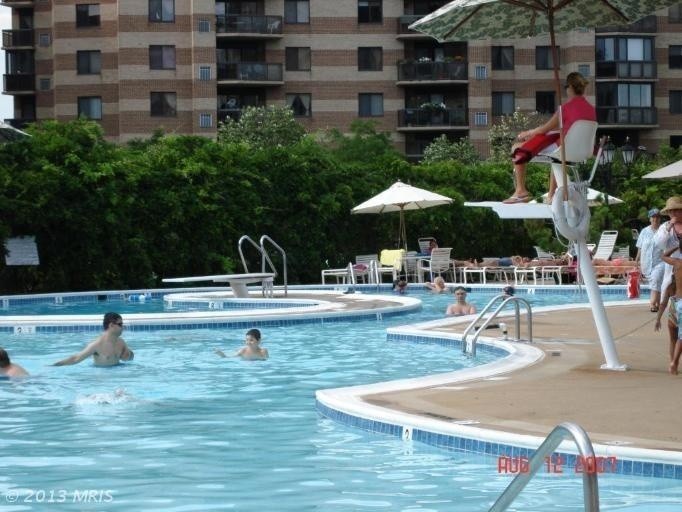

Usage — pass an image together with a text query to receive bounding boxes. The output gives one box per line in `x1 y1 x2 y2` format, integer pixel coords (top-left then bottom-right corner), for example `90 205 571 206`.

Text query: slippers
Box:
503 195 533 204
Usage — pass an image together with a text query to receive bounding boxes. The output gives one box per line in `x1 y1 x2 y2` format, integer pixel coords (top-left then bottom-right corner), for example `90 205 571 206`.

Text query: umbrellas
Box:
409 1 682 199
350 179 453 252
529 182 623 206
642 161 682 180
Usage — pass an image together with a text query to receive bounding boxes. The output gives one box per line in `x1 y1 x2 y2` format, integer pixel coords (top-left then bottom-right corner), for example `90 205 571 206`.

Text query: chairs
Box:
320 226 634 287
512 118 599 200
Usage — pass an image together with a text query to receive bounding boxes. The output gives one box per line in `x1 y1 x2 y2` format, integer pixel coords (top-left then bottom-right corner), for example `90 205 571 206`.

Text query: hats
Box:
660 196 682 215
648 209 659 216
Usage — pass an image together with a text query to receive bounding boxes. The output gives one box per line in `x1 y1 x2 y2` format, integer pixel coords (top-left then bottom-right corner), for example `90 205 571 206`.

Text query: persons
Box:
632 196 682 373
0 347 27 380
593 257 639 265
49 311 134 366
390 236 580 315
217 330 268 360
502 73 597 203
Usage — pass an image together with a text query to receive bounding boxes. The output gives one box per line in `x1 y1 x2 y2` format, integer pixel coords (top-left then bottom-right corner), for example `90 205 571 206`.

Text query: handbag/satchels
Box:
627 269 640 299
